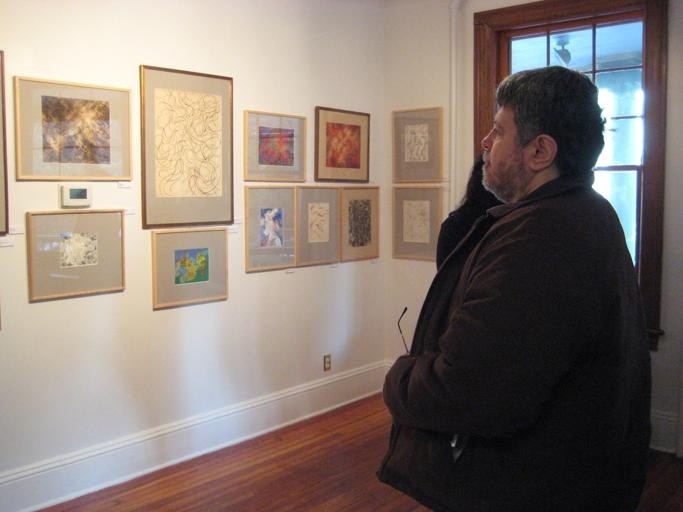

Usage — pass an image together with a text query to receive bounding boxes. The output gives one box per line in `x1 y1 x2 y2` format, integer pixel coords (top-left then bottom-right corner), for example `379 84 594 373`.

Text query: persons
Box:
436 154 502 272
374 67 651 512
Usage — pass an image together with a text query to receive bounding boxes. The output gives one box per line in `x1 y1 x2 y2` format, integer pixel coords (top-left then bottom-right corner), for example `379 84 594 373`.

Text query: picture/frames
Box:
388 180 444 264
136 62 236 231
12 75 134 183
311 104 371 185
149 227 229 312
0 49 10 237
241 181 380 274
241 108 308 184
388 103 443 184
23 208 125 304
